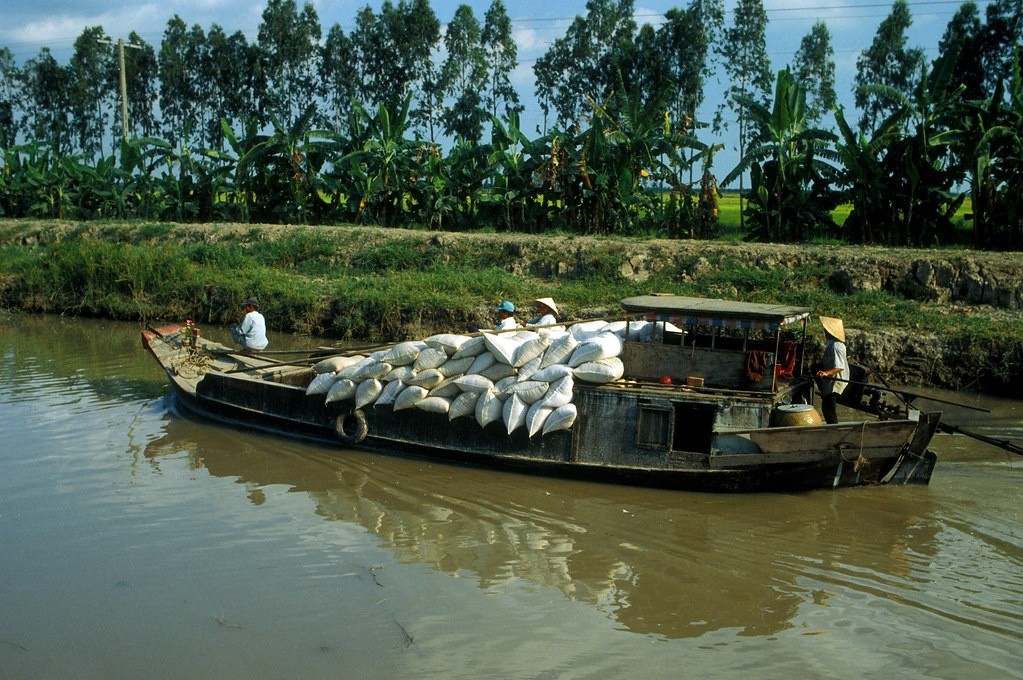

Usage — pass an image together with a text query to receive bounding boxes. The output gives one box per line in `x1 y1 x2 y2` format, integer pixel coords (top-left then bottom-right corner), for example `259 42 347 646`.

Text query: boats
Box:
141 292 947 496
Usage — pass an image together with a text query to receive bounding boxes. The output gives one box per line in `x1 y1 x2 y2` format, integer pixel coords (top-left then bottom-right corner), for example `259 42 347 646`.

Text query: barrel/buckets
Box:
776 404 822 427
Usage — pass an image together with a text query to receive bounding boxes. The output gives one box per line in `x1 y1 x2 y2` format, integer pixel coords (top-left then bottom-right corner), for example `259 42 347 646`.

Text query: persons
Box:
469 301 516 333
520 298 561 331
230 297 269 352
815 316 850 424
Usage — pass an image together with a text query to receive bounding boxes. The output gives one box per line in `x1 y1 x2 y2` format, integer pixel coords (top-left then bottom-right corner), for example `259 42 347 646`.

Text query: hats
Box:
534 298 559 315
495 301 514 312
819 315 845 342
241 297 259 306
